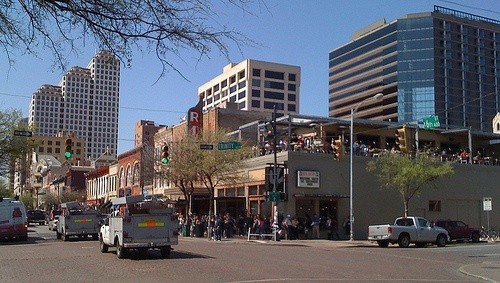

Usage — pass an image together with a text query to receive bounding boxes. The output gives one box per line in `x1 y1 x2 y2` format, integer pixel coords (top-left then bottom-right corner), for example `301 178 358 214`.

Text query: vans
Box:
0 197 27 242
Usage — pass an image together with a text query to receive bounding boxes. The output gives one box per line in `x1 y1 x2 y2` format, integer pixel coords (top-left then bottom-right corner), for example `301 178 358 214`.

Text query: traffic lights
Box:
160 144 169 164
331 136 343 161
64 138 72 160
394 123 409 161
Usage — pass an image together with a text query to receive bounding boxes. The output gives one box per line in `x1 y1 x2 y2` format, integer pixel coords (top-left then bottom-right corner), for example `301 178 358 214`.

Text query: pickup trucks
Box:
367 216 450 248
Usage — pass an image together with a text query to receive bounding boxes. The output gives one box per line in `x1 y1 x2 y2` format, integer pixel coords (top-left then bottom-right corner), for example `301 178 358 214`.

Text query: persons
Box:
344 139 400 158
253 137 288 157
290 133 334 155
177 212 209 238
435 147 500 165
276 213 340 240
211 211 272 242
343 216 354 239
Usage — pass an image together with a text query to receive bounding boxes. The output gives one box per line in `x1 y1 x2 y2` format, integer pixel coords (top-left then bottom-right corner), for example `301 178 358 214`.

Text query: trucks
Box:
54 201 100 241
99 194 180 258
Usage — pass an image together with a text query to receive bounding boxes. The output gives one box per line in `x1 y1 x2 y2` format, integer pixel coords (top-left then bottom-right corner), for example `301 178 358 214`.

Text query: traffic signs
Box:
14 130 32 137
200 144 213 150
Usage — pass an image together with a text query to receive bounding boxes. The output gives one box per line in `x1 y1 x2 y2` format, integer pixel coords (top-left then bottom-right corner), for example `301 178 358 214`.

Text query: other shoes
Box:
214 240 220 241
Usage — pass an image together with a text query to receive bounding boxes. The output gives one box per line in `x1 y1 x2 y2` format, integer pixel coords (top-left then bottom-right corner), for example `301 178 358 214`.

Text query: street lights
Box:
262 105 277 242
349 93 384 241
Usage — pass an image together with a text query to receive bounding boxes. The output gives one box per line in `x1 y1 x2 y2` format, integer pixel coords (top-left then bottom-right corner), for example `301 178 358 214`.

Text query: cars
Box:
431 219 481 245
46 210 61 230
27 210 46 225
